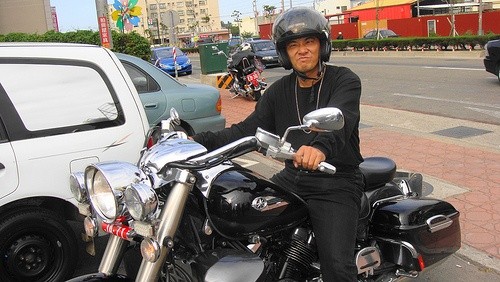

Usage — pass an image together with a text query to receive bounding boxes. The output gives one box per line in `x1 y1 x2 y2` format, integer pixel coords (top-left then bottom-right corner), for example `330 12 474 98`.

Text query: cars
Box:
149 46 192 77
482 40 500 85
113 51 226 137
245 39 283 68
361 29 399 39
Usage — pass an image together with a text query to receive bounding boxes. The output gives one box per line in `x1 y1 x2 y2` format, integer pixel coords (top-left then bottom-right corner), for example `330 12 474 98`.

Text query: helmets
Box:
272 6 332 70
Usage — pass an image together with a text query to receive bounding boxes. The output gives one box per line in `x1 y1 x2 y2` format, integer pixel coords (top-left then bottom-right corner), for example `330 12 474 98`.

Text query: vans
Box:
0 41 154 282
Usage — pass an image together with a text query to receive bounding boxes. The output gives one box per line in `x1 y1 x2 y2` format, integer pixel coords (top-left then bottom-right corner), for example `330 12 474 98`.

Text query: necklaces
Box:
294 76 324 134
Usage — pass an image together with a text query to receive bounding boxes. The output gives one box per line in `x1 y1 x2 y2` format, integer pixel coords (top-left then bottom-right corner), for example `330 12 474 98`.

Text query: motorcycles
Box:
211 41 268 101
60 106 463 282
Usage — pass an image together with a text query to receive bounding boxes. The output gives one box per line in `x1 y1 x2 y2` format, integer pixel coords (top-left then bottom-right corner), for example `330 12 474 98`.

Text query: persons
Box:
337 31 343 40
229 42 261 90
160 6 363 282
